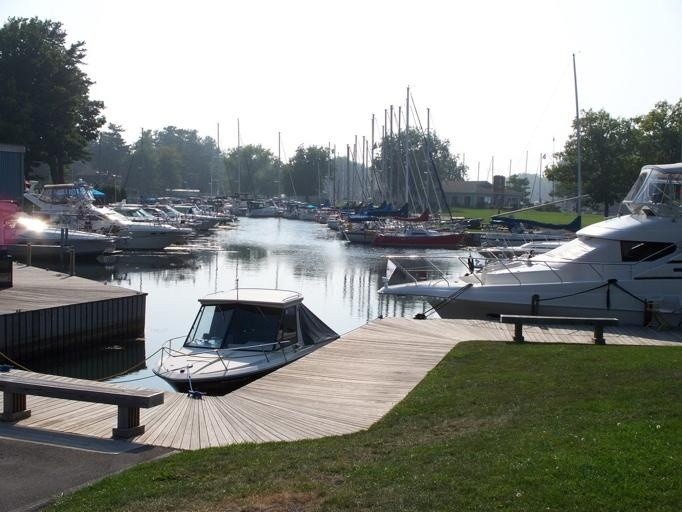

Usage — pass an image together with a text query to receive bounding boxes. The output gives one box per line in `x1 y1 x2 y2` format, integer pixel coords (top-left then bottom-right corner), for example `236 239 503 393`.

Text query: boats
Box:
0 176 237 260
153 286 341 395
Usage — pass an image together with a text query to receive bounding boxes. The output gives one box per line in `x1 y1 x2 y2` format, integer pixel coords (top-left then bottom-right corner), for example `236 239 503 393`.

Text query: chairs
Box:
644 298 676 332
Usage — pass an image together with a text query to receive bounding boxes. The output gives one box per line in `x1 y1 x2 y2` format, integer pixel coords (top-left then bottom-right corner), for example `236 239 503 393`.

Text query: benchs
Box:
501 314 618 345
0 369 164 439
